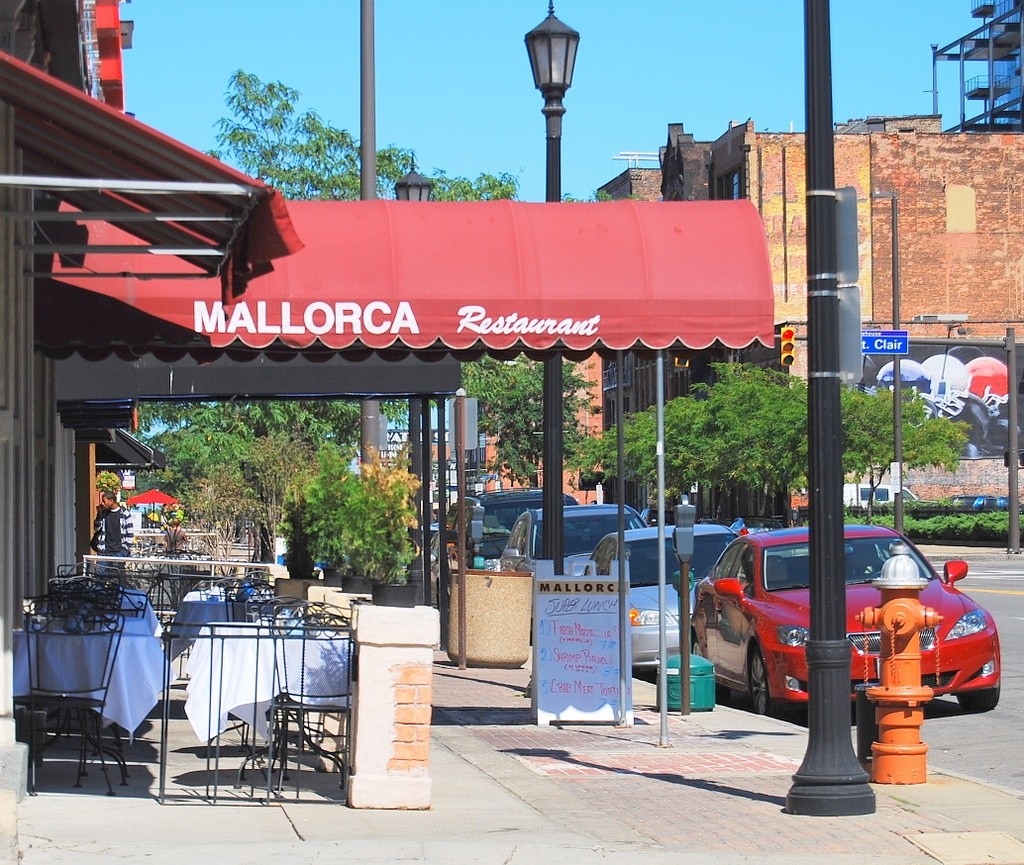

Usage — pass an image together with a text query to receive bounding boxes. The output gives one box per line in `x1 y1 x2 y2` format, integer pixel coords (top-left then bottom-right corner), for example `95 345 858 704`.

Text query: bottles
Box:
276 608 292 636
237 581 254 603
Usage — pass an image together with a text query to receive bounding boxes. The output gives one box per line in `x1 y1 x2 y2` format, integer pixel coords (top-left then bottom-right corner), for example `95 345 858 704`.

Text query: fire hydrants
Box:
855 543 946 785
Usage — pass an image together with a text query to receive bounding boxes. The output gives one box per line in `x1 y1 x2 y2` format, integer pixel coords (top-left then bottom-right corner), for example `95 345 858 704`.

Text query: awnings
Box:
1 51 308 321
36 201 775 361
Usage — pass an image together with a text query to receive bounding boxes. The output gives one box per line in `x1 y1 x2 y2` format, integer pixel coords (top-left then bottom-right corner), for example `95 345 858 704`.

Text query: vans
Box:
843 483 920 508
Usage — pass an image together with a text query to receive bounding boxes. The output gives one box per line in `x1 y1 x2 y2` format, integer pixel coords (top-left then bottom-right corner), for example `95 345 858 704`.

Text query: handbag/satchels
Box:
90 531 101 552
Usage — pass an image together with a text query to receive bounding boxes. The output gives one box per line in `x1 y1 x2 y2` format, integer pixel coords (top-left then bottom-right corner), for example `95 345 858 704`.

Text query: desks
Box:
12 630 162 789
93 586 164 637
184 620 355 790
168 590 276 663
35 616 151 634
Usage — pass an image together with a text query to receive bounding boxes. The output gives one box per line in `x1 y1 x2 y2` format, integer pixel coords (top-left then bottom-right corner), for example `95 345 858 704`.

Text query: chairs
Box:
852 542 884 579
766 555 789 588
21 550 354 806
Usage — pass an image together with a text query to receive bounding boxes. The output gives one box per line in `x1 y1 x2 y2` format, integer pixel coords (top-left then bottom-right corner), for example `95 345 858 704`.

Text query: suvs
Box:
501 504 651 647
431 487 579 611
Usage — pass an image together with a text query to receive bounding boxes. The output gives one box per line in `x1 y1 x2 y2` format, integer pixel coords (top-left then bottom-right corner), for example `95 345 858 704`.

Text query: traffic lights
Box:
780 326 796 366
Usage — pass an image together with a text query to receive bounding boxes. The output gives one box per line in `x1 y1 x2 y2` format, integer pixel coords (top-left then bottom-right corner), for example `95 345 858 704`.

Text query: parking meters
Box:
468 499 487 557
789 507 801 528
670 494 696 717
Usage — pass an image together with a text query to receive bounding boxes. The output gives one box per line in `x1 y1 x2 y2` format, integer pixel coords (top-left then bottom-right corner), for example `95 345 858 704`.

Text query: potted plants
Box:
277 441 420 608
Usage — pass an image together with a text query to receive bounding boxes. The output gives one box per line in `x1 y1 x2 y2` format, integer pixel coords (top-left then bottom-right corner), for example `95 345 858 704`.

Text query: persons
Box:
93 492 134 587
148 510 158 527
163 518 188 598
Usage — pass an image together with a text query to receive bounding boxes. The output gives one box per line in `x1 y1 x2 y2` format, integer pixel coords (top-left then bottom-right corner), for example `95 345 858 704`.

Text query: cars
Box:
689 523 1002 718
640 507 675 528
943 494 1024 514
727 516 786 547
584 524 741 670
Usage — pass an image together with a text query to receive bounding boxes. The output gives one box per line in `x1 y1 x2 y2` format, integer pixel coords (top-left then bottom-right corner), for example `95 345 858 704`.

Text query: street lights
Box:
868 189 904 539
524 0 582 699
393 151 434 606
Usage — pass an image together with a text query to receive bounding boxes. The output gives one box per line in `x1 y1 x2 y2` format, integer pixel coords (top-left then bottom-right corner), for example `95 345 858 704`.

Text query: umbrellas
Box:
126 489 179 528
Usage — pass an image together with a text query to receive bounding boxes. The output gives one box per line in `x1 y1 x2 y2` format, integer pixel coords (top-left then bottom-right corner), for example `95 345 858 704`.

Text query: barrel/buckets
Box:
659 655 715 712
